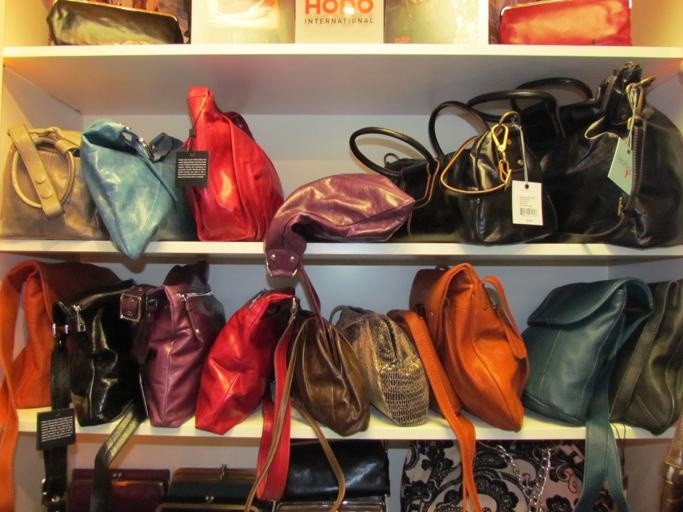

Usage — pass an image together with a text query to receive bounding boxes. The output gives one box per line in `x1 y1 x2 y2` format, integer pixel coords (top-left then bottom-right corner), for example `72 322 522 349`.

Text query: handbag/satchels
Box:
285 438 385 498
246 314 371 512
388 311 481 512
662 412 683 512
498 0 632 45
10 125 105 239
400 441 627 512
185 87 283 243
117 262 226 427
330 307 429 428
83 121 192 260
520 278 655 511
409 262 529 432
47 0 184 45
194 287 299 502
38 279 138 512
350 62 559 244
466 62 683 249
609 278 683 435
0 259 121 512
265 176 416 332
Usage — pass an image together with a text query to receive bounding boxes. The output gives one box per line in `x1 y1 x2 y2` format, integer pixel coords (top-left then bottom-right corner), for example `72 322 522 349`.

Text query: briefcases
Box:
68 465 168 510
157 464 268 512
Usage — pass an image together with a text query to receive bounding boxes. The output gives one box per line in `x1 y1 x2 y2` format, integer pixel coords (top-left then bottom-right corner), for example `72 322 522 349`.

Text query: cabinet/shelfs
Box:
0 45 683 512
